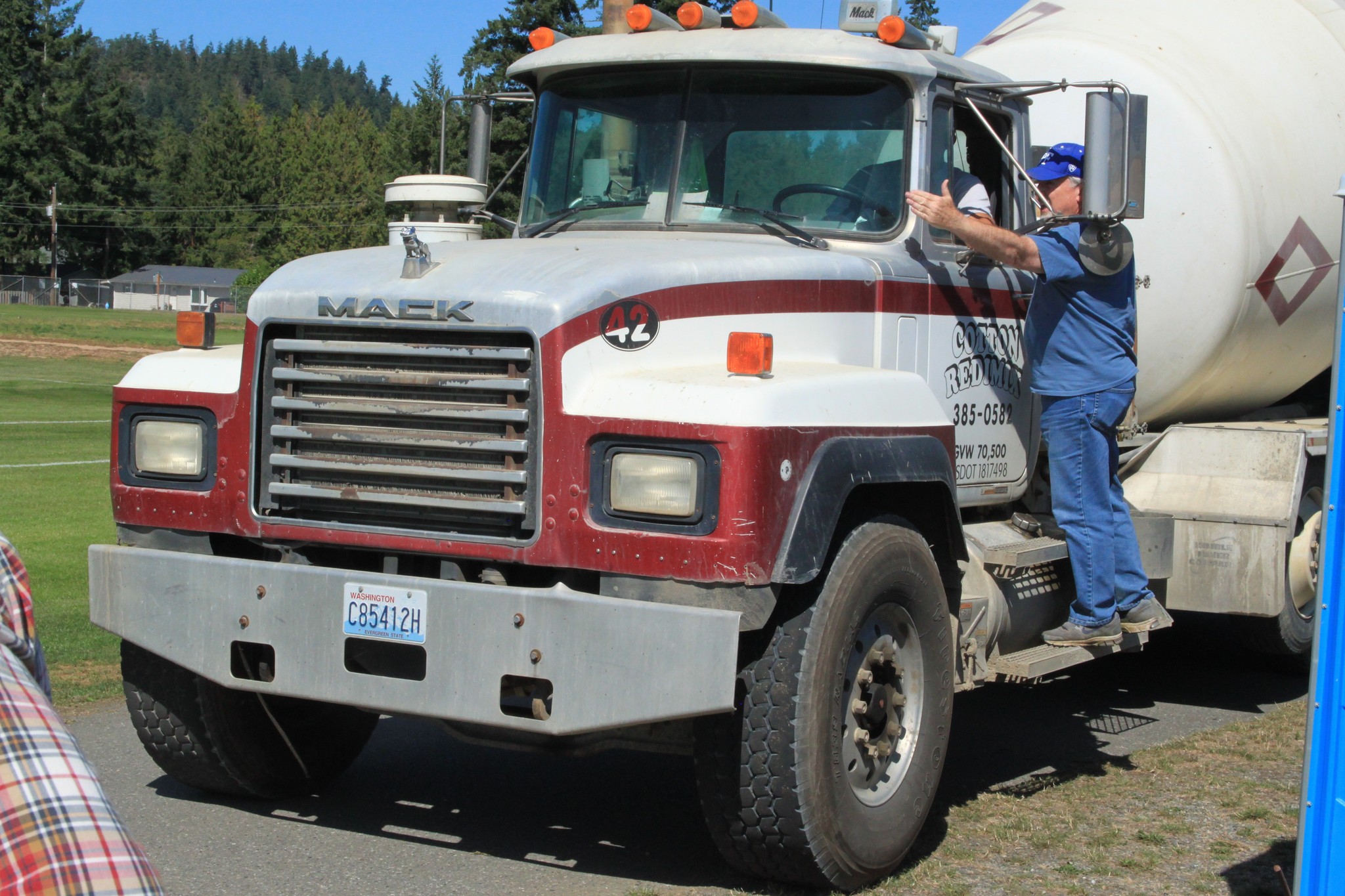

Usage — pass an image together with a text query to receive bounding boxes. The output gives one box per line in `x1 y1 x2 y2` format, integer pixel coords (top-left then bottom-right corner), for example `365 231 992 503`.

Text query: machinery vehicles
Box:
86 0 1344 895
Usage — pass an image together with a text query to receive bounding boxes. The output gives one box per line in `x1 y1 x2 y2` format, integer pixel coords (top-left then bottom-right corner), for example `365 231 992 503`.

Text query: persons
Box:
0 533 161 896
820 92 993 244
907 146 1176 641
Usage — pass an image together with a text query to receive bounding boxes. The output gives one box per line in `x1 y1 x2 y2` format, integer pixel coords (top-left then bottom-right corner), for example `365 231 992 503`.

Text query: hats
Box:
1017 143 1085 181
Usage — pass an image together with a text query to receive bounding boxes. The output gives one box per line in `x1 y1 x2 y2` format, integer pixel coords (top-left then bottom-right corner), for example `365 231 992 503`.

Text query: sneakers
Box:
1120 597 1161 634
1039 611 1123 649
